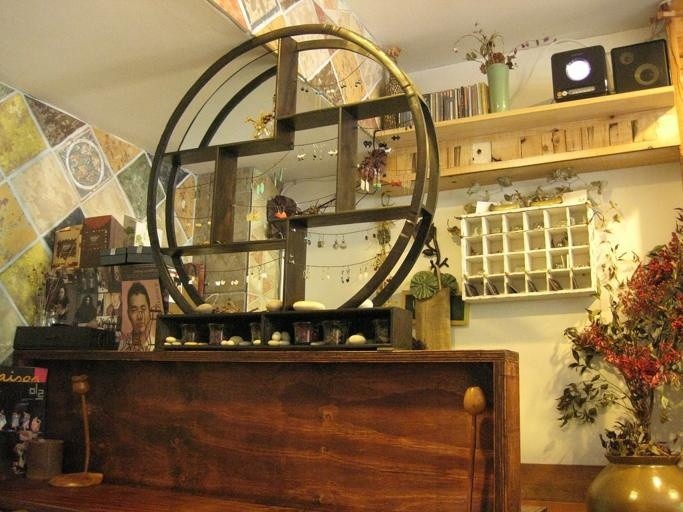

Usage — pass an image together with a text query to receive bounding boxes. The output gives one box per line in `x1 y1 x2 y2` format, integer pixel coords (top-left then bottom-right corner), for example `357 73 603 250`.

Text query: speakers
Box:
611 38 671 95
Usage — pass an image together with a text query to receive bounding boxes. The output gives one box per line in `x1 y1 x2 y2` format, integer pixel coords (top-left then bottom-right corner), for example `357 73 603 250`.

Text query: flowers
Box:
560 205 683 454
452 20 558 73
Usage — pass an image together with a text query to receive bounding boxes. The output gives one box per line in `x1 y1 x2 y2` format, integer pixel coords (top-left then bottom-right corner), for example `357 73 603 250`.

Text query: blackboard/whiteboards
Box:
0 365 49 481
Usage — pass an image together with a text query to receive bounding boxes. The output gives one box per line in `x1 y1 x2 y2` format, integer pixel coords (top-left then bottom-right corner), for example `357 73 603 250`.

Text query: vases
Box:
486 63 510 114
583 456 683 512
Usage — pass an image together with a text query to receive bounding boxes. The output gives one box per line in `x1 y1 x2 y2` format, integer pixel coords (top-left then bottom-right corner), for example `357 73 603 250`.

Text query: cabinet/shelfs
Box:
455 198 600 302
373 81 683 196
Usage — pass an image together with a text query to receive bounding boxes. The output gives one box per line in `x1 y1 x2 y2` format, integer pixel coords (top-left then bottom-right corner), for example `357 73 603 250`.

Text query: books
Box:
400 83 489 127
0 366 48 478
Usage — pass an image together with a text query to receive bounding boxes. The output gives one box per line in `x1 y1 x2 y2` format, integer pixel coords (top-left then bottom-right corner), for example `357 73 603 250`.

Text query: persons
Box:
120 282 155 352
